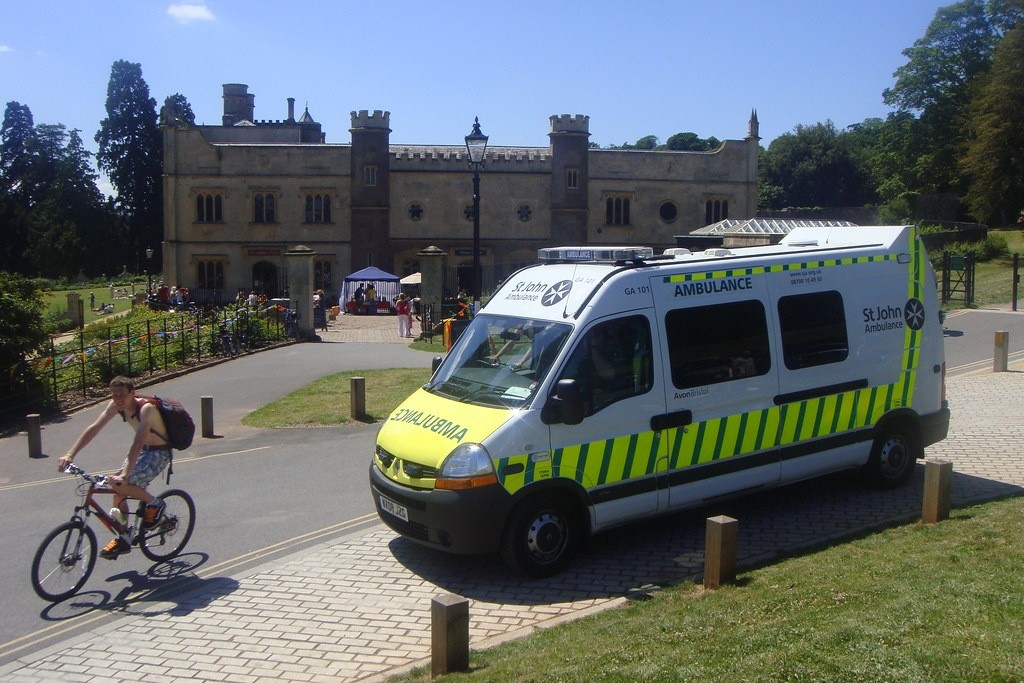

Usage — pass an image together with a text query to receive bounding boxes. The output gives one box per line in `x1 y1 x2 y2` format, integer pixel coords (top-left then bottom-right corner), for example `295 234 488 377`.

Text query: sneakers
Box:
97 536 131 558
142 497 166 530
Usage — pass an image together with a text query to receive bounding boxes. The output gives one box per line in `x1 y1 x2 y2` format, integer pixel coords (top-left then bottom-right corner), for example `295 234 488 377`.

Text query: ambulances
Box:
368 225 951 579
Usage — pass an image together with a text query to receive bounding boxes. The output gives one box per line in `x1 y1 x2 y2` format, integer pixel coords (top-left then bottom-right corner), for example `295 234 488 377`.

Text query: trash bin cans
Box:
443 319 476 352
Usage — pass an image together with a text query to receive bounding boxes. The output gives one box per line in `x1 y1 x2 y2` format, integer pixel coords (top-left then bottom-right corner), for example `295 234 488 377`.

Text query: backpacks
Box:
118 393 196 451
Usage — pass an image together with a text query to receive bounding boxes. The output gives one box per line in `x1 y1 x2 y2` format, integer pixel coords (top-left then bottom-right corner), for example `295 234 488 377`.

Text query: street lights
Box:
465 115 490 303
145 245 155 299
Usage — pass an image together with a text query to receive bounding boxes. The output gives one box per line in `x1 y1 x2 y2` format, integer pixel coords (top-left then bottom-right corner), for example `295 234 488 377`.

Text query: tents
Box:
341 266 401 314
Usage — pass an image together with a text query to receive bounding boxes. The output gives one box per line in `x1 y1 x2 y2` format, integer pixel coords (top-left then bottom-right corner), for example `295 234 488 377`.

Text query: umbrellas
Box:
399 271 421 292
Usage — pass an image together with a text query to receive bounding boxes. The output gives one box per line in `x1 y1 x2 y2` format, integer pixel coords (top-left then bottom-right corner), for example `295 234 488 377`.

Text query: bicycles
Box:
30 462 197 603
217 307 301 357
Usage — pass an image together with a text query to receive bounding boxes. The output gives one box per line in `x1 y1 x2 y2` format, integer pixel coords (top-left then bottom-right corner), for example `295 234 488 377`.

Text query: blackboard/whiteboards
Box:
314 308 327 329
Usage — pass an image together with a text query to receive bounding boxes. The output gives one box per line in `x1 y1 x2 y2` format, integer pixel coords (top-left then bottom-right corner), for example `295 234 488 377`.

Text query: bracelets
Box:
65 453 74 460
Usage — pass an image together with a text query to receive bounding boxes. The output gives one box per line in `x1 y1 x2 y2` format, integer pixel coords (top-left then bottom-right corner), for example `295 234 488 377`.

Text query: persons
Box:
578 323 636 390
235 288 268 308
96 302 114 316
109 283 128 300
283 285 290 298
88 292 95 312
490 323 545 368
354 282 376 304
131 283 136 294
146 276 190 311
393 293 421 338
497 279 503 288
422 312 431 332
58 376 195 559
457 287 474 309
312 289 327 308
